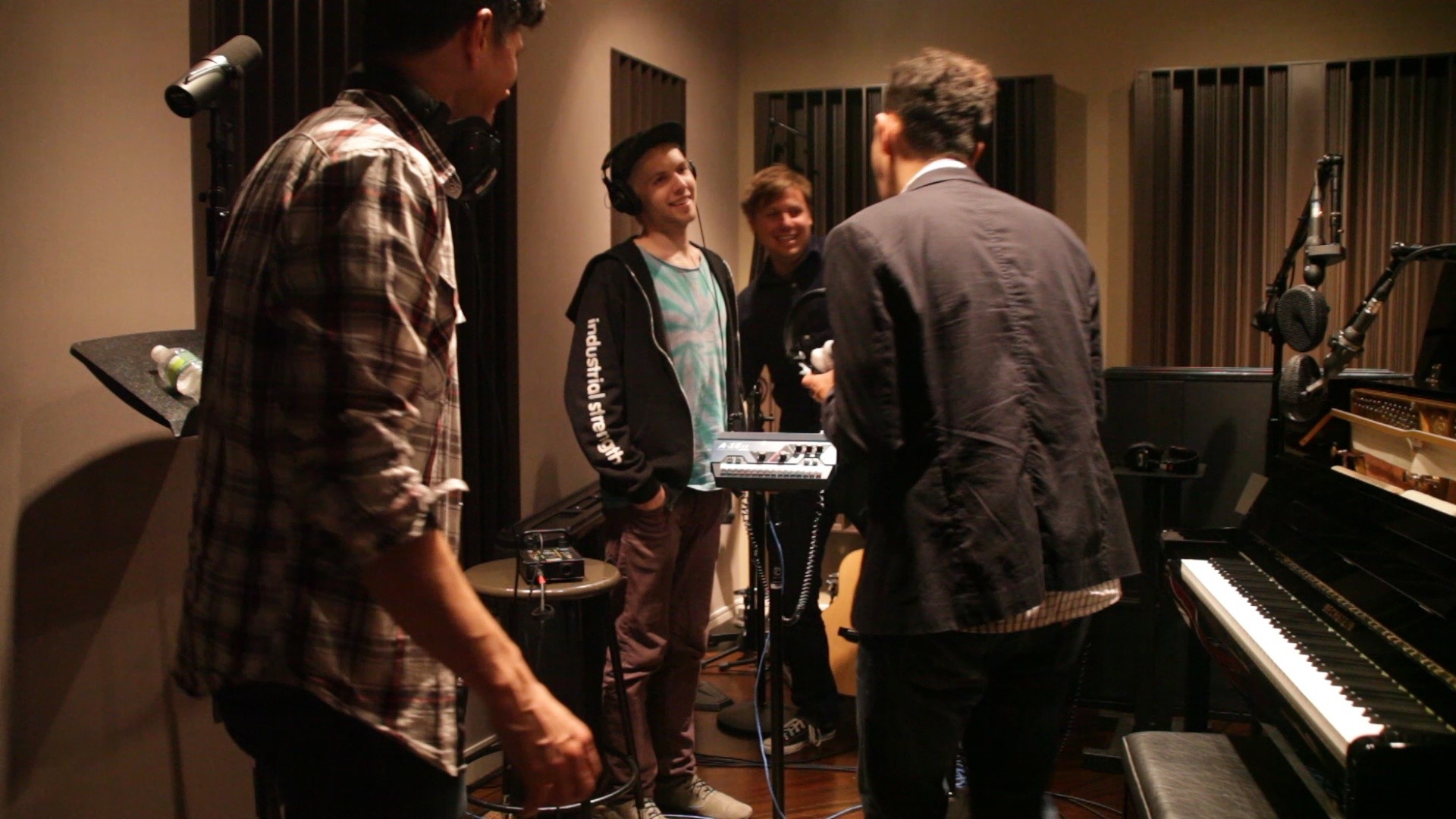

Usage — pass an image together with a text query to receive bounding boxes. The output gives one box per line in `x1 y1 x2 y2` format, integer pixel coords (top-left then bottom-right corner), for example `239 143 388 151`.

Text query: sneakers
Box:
762 718 837 755
592 774 753 819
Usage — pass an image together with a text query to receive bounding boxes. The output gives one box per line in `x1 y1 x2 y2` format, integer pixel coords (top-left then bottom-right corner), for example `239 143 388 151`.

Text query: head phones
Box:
601 136 696 215
782 287 838 378
1124 443 1202 476
363 69 505 201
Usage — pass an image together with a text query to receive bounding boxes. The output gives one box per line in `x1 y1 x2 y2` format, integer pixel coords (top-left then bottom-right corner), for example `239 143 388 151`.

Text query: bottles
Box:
151 344 203 403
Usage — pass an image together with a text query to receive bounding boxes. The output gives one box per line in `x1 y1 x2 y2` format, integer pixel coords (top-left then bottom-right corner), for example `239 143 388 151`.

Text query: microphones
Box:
1321 277 1398 378
165 35 263 118
769 117 806 139
1303 185 1327 285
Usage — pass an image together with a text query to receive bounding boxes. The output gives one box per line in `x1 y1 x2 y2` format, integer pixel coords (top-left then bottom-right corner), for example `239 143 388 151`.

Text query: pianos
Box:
1170 376 1456 819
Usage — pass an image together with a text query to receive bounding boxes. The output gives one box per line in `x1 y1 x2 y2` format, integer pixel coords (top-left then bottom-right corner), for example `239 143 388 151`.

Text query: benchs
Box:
1120 731 1280 818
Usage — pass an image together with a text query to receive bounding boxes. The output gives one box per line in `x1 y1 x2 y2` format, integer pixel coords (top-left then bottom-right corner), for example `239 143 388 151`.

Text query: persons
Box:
802 45 1139 819
731 166 848 759
564 122 757 819
173 0 599 819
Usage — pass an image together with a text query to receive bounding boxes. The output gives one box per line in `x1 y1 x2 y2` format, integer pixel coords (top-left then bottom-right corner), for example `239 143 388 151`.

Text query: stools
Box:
453 556 647 819
